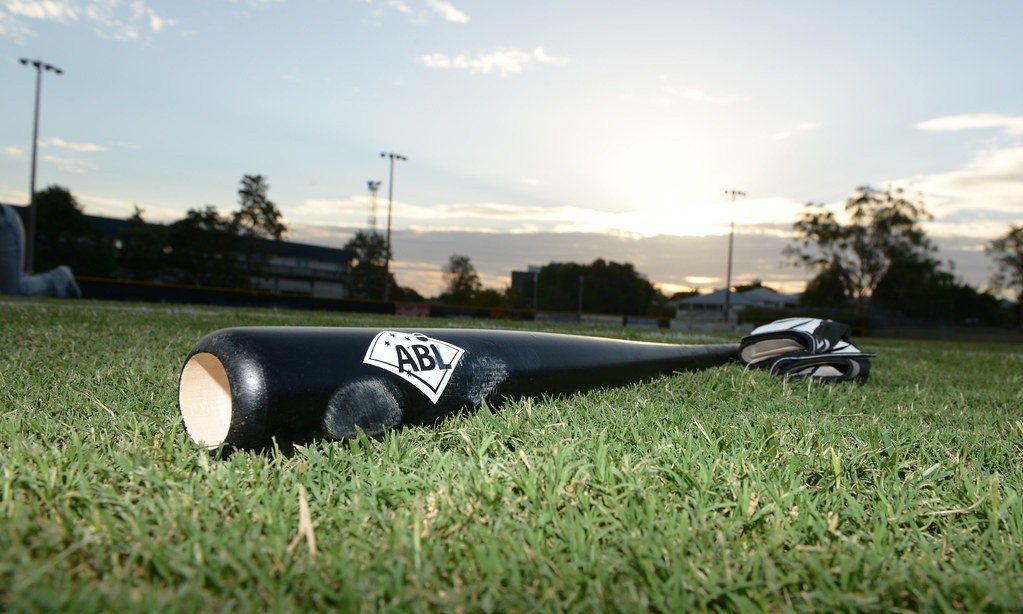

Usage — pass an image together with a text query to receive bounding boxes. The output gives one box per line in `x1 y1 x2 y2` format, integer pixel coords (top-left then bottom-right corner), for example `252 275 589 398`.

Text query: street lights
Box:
382 153 407 297
18 57 62 269
723 190 745 320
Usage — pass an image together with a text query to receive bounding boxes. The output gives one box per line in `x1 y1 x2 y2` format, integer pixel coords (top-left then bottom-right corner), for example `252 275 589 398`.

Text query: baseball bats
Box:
174 323 745 460
